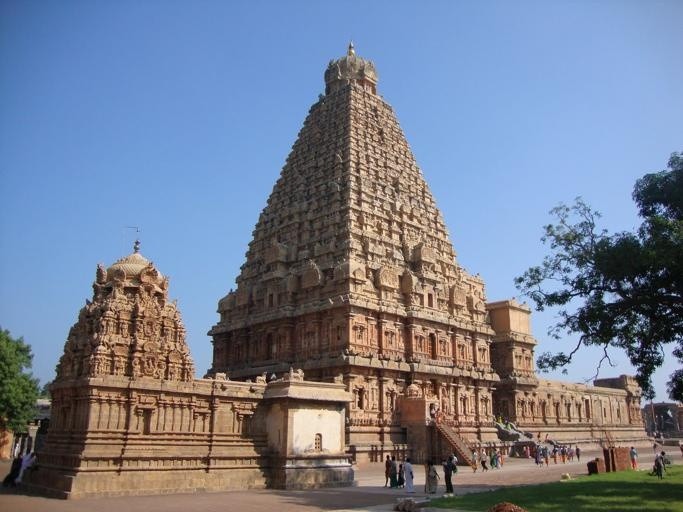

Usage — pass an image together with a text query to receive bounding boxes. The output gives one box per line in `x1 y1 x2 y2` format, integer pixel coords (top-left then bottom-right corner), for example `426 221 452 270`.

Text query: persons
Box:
655 451 666 479
382 405 583 499
646 429 671 454
3 439 36 488
630 447 639 470
648 460 658 476
